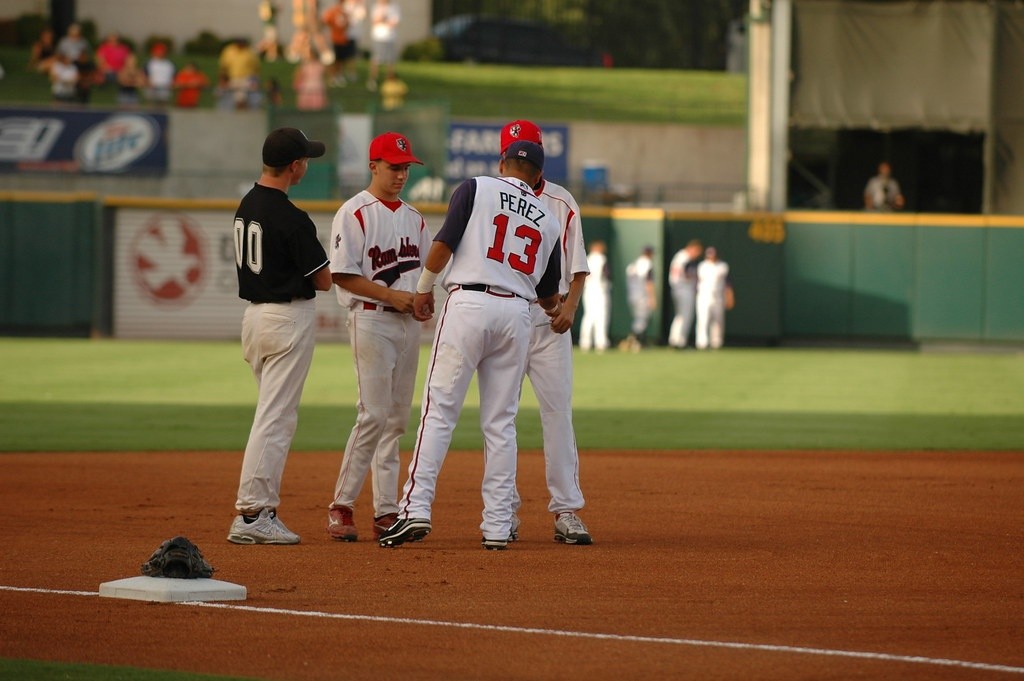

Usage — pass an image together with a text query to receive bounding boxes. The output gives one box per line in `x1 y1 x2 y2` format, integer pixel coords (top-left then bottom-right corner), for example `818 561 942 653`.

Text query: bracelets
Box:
545 303 558 314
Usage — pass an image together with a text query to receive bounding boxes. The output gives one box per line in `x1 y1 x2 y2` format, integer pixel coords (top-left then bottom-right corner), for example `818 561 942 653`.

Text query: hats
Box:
499 120 542 155
369 131 424 165
262 127 325 167
505 141 544 171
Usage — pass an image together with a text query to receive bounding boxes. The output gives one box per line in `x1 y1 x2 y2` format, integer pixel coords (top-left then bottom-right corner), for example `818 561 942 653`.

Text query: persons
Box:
668 238 734 349
329 131 432 542
28 0 409 111
579 241 613 351
864 163 904 211
500 119 593 545
378 141 562 551
226 127 332 544
626 243 657 347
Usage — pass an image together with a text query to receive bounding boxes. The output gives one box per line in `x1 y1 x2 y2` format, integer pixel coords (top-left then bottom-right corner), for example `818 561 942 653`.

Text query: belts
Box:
462 284 530 302
363 302 399 312
251 298 291 304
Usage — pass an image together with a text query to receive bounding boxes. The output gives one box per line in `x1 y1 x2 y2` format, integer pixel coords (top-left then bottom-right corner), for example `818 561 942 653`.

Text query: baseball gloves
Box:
140 536 215 579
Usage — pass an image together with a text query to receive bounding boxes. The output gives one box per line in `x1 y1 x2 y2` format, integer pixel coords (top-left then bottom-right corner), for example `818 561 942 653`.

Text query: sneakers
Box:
508 514 520 542
380 518 432 547
326 507 359 540
481 536 507 550
553 513 593 544
226 508 300 545
373 514 422 543
